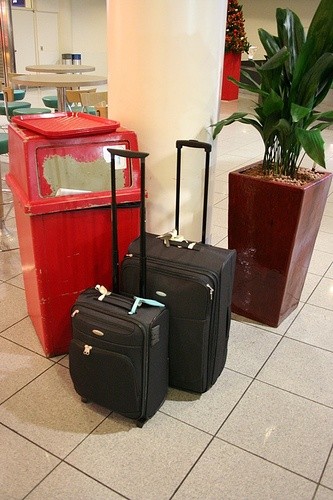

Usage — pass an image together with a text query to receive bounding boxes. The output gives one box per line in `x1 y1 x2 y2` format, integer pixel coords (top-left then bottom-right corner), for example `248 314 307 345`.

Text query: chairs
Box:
2 86 51 123
65 88 96 116
0 73 31 101
78 92 108 117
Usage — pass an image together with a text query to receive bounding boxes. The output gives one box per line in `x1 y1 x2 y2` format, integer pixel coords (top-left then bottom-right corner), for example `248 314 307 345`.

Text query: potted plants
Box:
208 0 333 328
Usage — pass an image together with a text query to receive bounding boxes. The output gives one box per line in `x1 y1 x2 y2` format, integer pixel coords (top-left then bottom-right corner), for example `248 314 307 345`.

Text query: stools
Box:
0 100 31 116
43 95 72 107
0 129 15 230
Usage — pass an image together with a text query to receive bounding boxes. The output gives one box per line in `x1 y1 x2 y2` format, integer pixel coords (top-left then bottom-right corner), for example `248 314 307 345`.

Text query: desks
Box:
61 53 82 65
25 64 95 74
12 74 107 112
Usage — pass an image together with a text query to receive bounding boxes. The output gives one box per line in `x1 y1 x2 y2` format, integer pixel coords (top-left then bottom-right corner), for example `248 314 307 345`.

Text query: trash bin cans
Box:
4 121 147 359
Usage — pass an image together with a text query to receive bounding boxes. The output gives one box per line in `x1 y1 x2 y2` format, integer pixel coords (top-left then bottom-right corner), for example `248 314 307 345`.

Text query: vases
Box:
221 51 241 100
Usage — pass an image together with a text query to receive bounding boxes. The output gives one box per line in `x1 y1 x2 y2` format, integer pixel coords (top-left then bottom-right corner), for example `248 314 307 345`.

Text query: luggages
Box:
69 149 169 428
121 139 237 394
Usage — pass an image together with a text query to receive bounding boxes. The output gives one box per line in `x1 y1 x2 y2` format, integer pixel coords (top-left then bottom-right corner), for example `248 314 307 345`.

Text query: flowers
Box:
225 0 252 54
